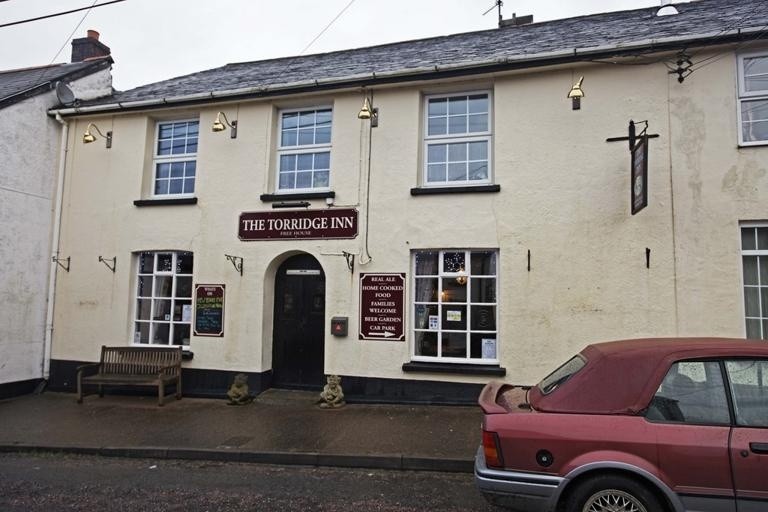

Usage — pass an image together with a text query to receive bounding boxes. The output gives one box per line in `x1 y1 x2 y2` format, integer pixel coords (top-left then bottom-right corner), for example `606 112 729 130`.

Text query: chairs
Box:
650 371 768 427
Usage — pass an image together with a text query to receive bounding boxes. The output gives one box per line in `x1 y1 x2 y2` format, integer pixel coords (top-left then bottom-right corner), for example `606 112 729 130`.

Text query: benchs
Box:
76 345 182 408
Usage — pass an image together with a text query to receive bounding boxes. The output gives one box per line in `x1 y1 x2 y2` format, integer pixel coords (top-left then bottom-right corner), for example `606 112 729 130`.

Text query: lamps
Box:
358 95 377 128
82 123 111 148
212 111 238 139
567 75 588 100
455 268 469 285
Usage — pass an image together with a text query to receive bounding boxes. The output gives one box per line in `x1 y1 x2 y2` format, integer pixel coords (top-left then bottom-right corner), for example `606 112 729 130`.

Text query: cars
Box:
472 334 768 512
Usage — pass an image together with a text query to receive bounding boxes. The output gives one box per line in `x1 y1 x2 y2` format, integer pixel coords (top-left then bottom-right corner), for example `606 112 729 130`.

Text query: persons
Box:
155 301 186 345
